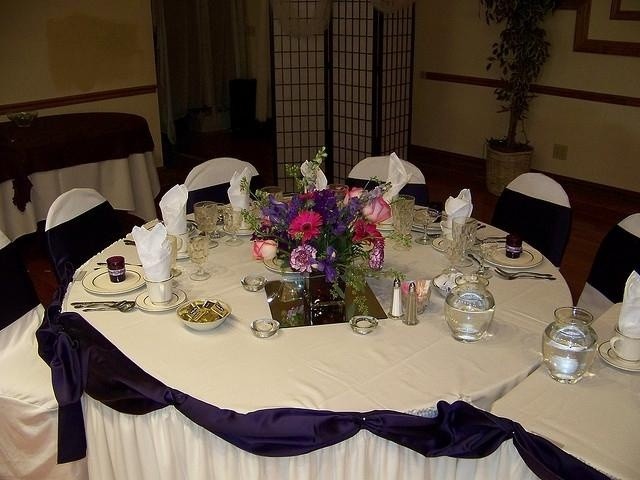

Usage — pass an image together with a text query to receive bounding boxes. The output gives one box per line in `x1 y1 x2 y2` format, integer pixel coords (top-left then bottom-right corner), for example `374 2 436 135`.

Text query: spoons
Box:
76 299 135 314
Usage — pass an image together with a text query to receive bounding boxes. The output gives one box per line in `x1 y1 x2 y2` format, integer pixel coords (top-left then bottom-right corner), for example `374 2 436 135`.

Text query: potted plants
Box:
476 0 567 198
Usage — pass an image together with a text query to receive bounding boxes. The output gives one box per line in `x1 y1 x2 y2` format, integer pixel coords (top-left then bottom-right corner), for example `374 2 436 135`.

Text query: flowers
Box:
237 152 395 322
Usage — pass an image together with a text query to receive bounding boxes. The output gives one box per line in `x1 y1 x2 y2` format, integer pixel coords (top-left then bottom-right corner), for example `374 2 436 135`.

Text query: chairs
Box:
0 148 640 479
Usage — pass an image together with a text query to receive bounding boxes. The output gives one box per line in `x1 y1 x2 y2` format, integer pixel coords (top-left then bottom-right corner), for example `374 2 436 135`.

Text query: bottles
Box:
386 277 405 322
443 274 497 343
536 306 597 384
403 282 422 326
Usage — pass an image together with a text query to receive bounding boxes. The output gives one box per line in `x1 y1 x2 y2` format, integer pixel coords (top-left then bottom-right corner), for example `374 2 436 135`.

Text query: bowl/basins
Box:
349 314 379 334
176 299 233 332
7 111 40 128
433 275 491 301
249 319 281 339
240 273 269 293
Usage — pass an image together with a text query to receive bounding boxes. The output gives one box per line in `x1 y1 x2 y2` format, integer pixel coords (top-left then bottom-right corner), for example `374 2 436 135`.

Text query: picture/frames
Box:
571 0 640 57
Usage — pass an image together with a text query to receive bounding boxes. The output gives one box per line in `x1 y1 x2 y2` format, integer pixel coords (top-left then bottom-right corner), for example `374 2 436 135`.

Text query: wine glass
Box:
389 193 418 251
189 236 213 281
476 242 499 279
221 206 246 246
412 206 441 246
193 201 218 251
448 215 478 268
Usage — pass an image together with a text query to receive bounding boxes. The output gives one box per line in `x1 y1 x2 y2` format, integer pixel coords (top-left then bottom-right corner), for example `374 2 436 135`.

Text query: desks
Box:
60 190 574 480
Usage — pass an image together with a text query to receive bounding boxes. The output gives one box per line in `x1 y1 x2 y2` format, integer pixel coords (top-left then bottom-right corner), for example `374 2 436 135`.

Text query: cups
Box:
327 184 349 202
263 186 285 203
164 228 190 277
608 325 640 362
506 233 524 259
143 275 174 304
107 256 126 283
402 288 430 315
215 202 224 225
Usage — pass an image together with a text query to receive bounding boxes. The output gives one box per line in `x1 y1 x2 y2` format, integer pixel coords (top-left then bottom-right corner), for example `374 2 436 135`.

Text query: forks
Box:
493 267 558 282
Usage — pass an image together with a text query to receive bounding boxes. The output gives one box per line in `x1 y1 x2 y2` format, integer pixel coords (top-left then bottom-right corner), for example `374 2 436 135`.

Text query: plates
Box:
430 237 448 252
370 219 444 235
81 265 149 295
133 289 188 312
261 257 300 275
225 224 258 236
479 241 544 268
597 339 640 372
144 219 200 238
